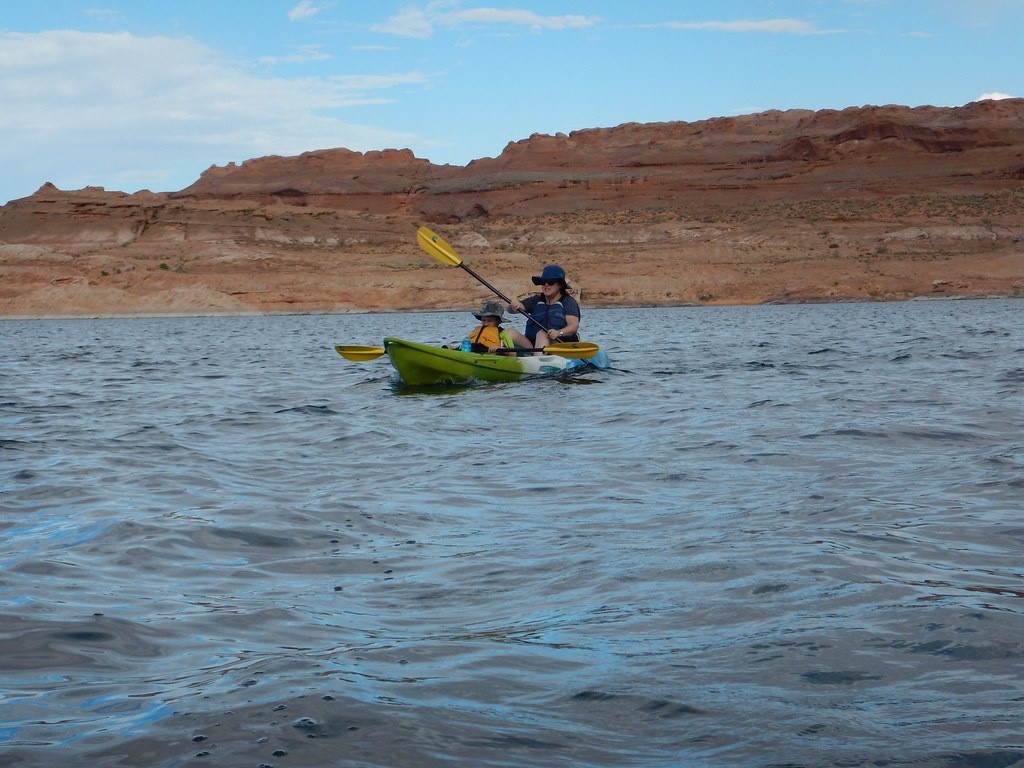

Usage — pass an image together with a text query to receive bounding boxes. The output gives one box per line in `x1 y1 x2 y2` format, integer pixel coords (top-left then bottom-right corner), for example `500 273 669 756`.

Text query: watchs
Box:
558 330 563 337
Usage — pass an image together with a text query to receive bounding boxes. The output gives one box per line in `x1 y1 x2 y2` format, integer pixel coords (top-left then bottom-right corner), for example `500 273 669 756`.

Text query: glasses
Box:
541 280 560 286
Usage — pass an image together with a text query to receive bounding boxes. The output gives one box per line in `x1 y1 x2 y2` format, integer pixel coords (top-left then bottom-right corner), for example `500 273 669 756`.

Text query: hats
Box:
532 265 565 285
471 301 511 323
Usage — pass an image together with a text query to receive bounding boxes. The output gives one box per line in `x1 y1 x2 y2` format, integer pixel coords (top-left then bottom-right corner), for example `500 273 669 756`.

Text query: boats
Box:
381 335 612 388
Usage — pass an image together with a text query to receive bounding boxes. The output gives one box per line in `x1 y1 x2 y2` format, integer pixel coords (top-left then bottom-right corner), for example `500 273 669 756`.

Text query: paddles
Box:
333 340 600 363
415 225 600 371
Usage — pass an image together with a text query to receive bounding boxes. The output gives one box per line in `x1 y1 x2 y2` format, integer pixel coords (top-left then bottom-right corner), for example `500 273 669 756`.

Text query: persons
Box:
507 265 581 357
441 301 516 356
